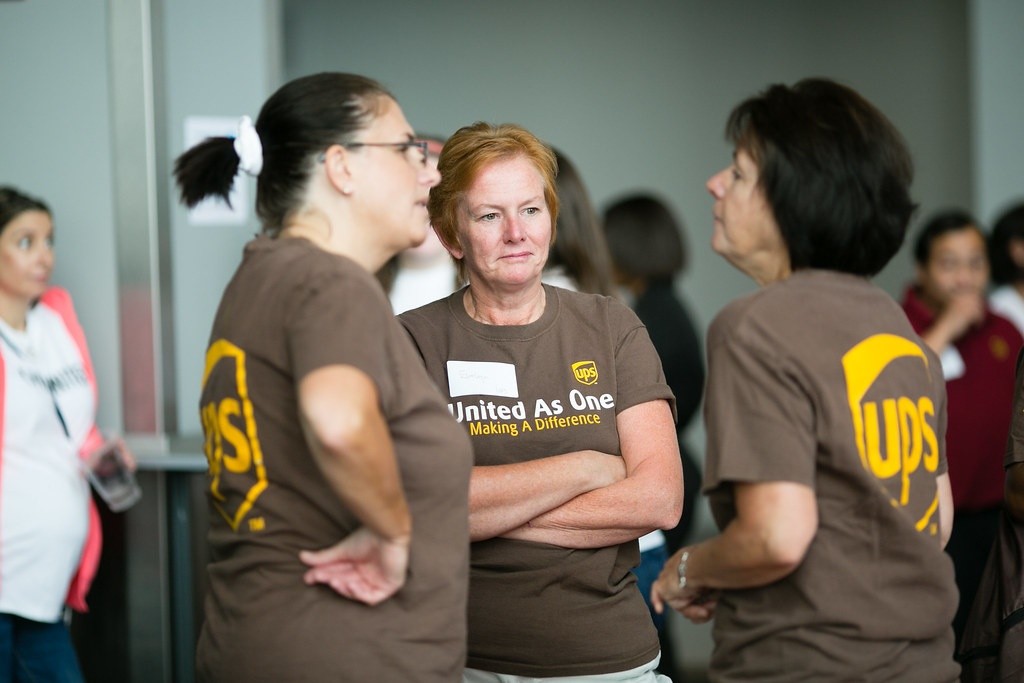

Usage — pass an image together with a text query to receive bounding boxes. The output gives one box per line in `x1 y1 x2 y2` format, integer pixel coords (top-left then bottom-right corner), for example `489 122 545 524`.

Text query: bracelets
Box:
676 540 693 597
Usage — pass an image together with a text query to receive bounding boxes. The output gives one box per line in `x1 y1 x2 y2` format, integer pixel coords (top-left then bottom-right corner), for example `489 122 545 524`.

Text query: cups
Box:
89 443 143 513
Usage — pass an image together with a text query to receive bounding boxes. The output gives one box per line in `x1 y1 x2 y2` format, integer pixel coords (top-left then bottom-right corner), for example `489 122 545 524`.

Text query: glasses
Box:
317 138 428 166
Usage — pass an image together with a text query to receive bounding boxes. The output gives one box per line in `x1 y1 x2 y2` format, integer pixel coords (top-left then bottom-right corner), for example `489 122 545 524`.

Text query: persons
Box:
173 68 464 683
0 185 141 683
382 78 1024 683
